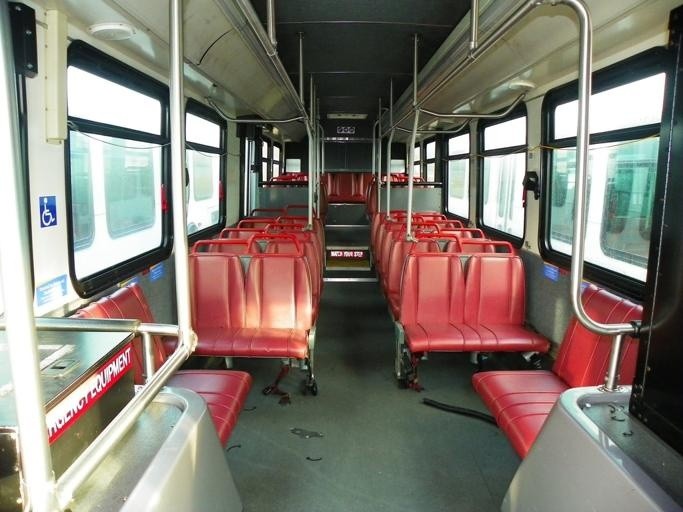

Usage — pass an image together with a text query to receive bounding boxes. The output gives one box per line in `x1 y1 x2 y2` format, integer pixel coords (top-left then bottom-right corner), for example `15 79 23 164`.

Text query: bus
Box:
66 64 219 281
400 73 669 283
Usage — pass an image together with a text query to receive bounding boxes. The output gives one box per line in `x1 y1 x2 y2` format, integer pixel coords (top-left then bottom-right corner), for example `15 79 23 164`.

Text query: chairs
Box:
268 171 427 211
188 203 324 396
369 210 550 388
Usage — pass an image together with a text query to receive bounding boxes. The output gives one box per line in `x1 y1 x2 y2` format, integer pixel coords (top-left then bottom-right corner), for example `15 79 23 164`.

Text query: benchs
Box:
472 285 644 461
69 281 250 450
